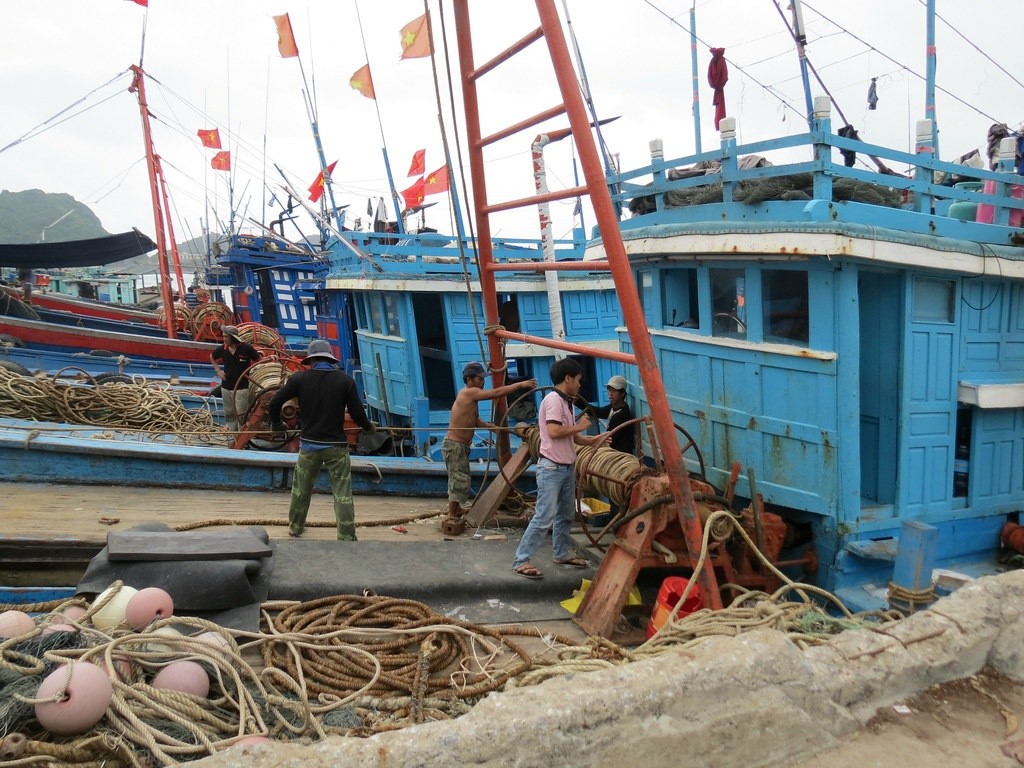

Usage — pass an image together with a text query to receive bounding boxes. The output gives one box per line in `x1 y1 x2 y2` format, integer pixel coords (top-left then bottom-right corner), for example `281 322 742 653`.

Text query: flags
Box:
407 150 425 177
273 14 299 58
123 0 148 7
198 129 222 149
211 151 230 171
400 165 449 210
307 160 337 203
350 64 376 100
400 10 435 59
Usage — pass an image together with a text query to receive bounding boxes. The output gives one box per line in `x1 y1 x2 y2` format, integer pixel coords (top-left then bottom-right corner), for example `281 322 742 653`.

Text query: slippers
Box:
511 563 544 578
553 558 585 568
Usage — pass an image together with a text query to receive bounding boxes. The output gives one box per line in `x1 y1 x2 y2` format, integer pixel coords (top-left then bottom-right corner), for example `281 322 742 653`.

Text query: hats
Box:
463 362 490 377
221 325 244 343
604 376 627 392
301 340 339 364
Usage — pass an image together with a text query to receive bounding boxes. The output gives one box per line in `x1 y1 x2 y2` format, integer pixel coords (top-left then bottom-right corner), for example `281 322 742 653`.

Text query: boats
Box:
0 0 1024 621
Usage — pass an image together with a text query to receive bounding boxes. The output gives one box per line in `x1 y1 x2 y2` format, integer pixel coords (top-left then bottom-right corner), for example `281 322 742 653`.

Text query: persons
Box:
441 361 538 518
511 358 612 579
210 324 280 432
269 340 379 541
184 287 200 309
573 376 634 455
